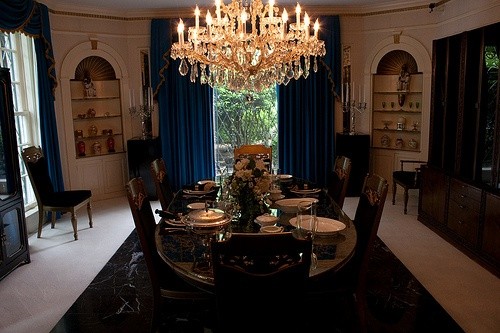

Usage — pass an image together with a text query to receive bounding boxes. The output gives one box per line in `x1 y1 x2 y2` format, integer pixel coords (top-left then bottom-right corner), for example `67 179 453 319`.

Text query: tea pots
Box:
254 213 279 226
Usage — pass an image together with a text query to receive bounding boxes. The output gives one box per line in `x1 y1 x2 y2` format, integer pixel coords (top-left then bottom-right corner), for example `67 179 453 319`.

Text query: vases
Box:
240 202 267 232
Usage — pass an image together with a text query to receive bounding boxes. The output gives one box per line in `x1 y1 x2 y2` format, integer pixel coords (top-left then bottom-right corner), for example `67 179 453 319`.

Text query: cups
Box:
260 226 284 232
269 189 282 199
408 141 416 149
220 176 230 200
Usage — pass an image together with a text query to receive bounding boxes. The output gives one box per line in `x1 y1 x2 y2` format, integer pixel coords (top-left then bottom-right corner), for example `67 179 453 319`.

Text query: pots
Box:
181 205 233 234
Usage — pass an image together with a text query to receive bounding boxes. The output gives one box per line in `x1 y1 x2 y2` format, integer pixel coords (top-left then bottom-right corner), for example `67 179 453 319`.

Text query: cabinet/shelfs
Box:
0 68 30 281
372 73 422 193
69 78 127 199
418 22 500 279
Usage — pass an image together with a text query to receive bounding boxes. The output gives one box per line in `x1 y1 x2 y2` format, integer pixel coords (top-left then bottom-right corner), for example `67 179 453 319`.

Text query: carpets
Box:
51 221 467 333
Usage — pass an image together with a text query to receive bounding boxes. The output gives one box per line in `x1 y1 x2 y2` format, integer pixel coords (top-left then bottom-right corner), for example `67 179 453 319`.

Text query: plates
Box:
279 174 292 179
164 202 210 227
269 187 346 235
183 180 215 194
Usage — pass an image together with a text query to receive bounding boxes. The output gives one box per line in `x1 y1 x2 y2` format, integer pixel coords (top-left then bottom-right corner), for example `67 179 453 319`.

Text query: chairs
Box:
332 158 387 333
124 159 211 333
392 160 428 215
234 144 273 175
23 146 93 240
210 233 311 333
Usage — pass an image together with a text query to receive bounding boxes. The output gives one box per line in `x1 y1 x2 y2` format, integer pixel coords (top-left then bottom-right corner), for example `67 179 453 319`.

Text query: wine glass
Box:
381 94 419 112
382 120 392 129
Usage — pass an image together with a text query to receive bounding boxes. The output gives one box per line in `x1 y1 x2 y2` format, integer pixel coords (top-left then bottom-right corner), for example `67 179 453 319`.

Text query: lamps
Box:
128 85 155 138
171 0 326 100
341 82 367 132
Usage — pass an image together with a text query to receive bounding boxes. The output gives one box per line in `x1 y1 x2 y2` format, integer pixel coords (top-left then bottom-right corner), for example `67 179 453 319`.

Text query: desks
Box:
335 130 370 196
126 138 160 201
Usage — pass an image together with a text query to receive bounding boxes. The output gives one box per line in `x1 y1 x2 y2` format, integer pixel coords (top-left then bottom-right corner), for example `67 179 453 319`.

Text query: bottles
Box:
395 138 403 148
89 124 97 136
107 137 114 152
87 108 96 118
78 141 85 154
93 142 101 155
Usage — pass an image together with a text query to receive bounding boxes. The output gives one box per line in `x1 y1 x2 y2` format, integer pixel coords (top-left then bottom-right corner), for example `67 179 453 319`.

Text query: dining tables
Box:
155 185 357 294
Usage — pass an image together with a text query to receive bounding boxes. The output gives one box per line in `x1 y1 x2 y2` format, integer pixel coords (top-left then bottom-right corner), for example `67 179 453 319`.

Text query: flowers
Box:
226 161 279 198
